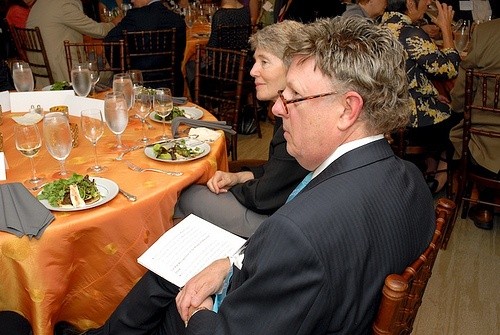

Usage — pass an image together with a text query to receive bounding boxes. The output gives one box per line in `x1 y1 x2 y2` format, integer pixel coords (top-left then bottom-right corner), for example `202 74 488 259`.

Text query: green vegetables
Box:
49 81 70 90
136 88 158 98
153 105 186 122
154 139 205 160
35 172 100 208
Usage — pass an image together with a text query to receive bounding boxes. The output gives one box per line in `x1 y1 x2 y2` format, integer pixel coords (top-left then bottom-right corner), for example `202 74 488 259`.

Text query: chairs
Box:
0 16 500 335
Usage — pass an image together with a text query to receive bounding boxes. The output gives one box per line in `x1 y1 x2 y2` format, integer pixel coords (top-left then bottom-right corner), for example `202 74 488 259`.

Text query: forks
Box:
126 161 183 178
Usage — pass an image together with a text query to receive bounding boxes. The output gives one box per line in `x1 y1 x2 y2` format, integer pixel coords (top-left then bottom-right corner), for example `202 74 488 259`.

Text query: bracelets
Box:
196 307 208 310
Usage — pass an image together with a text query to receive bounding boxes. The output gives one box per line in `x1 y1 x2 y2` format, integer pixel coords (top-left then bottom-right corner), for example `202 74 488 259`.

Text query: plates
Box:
145 142 210 163
194 33 210 38
149 107 204 124
37 177 120 211
42 82 72 91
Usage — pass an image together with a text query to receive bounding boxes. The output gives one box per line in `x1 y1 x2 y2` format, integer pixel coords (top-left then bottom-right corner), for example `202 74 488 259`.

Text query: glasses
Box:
278 90 366 114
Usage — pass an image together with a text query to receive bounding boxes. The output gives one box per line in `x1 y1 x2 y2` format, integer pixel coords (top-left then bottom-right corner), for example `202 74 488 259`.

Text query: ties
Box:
213 171 314 314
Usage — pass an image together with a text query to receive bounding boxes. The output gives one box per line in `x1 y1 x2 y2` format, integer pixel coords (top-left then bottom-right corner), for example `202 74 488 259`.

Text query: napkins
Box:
0 183 55 240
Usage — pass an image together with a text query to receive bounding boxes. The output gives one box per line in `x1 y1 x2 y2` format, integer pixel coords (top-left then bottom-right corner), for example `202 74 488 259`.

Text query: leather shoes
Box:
468 203 494 230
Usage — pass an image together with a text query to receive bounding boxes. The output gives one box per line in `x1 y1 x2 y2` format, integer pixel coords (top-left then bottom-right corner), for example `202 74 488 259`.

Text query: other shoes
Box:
54 321 82 335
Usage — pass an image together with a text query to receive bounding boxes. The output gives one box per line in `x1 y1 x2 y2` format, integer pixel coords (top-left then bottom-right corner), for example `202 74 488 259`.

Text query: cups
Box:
13 62 34 91
100 3 132 22
71 64 91 97
462 16 492 31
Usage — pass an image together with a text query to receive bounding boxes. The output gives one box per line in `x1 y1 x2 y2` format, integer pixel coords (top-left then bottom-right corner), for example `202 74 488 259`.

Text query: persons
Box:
53 13 436 335
279 0 500 198
0 0 289 97
172 19 307 237
25 0 124 87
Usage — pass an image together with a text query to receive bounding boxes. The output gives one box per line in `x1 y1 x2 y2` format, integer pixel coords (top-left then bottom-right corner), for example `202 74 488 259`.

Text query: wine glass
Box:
12 112 77 189
86 63 100 99
81 109 107 173
104 93 129 154
113 70 173 144
185 2 220 39
454 27 469 57
425 3 463 33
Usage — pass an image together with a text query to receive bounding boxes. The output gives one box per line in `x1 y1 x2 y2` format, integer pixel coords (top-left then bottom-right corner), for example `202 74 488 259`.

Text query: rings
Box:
179 285 184 291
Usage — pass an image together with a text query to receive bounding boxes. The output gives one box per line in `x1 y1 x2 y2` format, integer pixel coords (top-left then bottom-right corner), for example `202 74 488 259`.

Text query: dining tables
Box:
98 7 212 102
1 83 229 334
